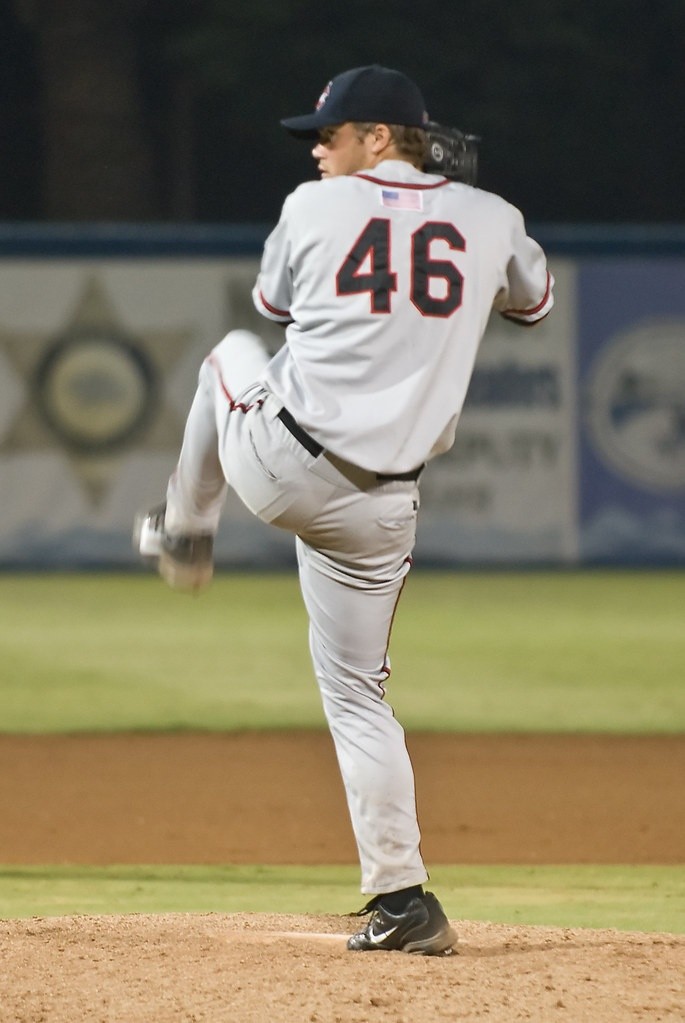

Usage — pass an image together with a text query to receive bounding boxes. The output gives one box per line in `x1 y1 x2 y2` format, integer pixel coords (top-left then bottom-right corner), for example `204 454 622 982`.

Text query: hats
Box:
282 64 432 136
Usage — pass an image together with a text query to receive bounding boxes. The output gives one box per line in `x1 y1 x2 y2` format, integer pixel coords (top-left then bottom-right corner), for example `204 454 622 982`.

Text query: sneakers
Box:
347 892 460 954
138 503 213 588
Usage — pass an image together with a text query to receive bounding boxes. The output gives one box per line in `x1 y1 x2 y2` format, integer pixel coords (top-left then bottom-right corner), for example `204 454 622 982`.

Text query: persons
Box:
138 65 557 952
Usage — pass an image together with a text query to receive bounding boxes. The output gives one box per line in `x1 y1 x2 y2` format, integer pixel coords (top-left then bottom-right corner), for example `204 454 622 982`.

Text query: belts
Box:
278 407 422 481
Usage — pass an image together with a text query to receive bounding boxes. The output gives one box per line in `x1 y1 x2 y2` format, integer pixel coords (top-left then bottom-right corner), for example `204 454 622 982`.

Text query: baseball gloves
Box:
425 122 483 186
